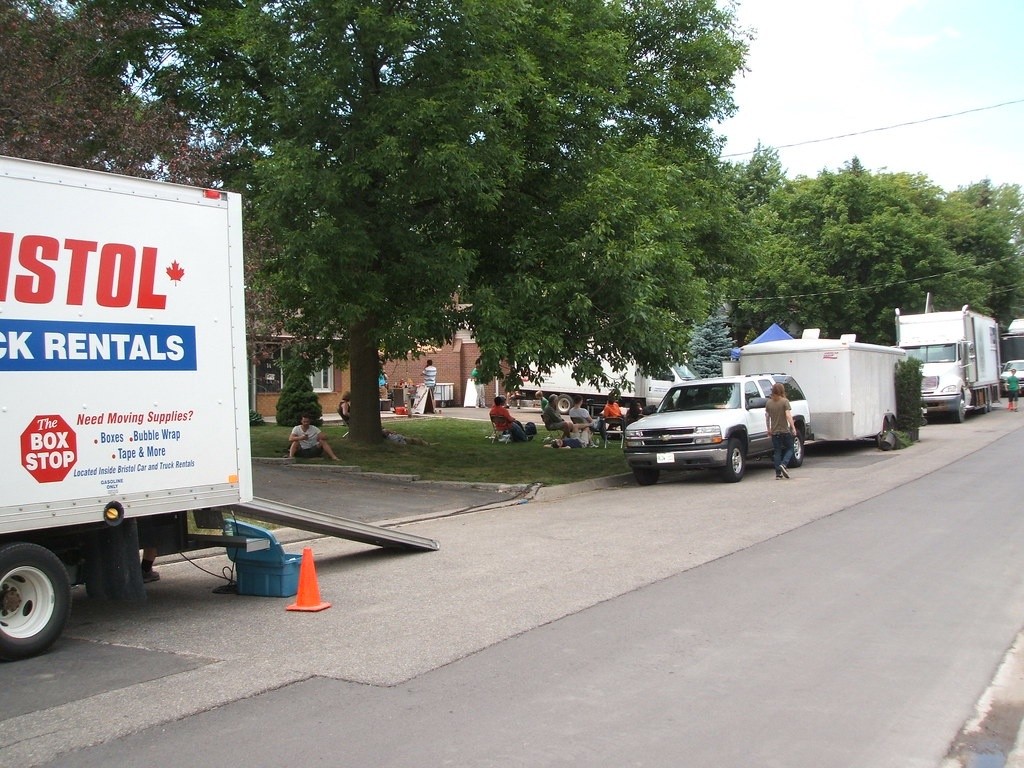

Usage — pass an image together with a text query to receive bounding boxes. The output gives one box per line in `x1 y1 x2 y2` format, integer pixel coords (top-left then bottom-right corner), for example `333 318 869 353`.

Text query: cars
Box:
1000 359 1024 398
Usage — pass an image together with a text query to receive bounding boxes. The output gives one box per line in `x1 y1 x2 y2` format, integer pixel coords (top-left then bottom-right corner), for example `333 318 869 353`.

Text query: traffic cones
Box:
286 546 331 611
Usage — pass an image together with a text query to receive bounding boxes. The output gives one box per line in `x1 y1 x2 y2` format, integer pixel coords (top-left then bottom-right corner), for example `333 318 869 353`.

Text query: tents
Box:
730 323 794 362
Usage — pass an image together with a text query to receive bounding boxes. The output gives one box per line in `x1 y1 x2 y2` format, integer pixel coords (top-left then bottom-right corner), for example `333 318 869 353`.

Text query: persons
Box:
604 393 622 430
569 395 611 444
472 365 488 408
765 383 797 480
626 397 641 422
383 428 440 446
1004 369 1021 411
379 361 388 386
535 391 548 410
503 367 520 409
339 391 350 418
420 360 437 400
289 415 340 461
543 394 573 439
141 547 160 583
490 395 534 441
543 427 599 449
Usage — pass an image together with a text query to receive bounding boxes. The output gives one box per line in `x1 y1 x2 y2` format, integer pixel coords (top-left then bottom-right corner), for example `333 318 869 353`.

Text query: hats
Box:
499 395 506 402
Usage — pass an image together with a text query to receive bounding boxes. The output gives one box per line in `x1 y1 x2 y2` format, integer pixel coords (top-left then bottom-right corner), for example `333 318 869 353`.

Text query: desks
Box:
603 431 625 449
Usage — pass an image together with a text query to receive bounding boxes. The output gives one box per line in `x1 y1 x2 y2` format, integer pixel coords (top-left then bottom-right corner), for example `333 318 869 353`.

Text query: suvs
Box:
623 371 815 487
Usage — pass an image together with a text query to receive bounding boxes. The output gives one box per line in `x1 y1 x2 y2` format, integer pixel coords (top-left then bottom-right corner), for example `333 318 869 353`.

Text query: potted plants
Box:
880 357 927 450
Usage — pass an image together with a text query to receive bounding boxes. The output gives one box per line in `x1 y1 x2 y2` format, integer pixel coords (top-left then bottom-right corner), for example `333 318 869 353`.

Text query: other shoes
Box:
776 476 780 480
780 463 790 480
1014 408 1017 412
604 439 611 443
528 436 534 441
476 405 479 408
590 444 599 448
142 567 160 583
482 405 488 407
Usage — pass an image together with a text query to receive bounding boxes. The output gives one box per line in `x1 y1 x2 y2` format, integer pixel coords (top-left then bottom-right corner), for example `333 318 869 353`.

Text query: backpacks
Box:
525 422 536 435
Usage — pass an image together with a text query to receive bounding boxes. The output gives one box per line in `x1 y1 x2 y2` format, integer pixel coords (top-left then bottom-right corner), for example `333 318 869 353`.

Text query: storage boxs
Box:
226 520 303 598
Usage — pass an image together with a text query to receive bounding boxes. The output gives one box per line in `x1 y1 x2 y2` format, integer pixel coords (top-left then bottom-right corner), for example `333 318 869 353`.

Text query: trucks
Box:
0 156 256 664
892 291 1003 423
999 318 1024 372
508 337 704 416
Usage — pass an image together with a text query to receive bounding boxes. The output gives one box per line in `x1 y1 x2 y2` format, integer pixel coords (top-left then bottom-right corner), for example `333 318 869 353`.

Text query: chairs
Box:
541 414 562 441
489 416 513 444
335 407 350 438
603 417 626 438
